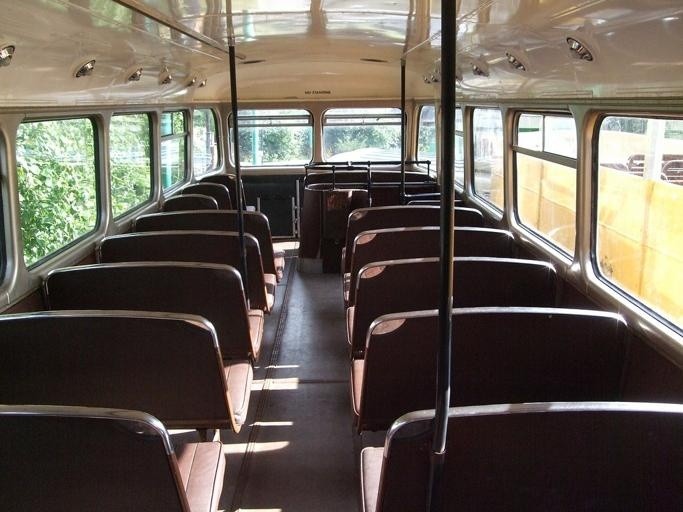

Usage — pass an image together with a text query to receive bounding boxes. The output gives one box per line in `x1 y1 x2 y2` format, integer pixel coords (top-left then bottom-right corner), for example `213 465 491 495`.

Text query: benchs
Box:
0 162 683 512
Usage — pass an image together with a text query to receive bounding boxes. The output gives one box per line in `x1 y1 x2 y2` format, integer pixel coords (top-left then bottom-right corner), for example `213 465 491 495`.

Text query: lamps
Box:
423 36 595 83
0 44 208 88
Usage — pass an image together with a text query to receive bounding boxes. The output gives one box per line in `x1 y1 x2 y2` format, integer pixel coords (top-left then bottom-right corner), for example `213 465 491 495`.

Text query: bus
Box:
489 110 683 325
